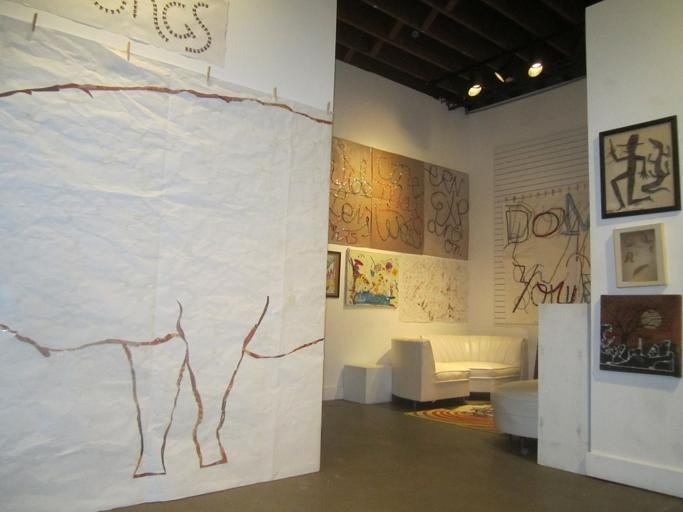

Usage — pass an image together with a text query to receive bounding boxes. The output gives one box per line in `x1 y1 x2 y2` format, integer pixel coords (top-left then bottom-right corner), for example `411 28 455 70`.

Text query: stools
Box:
489 376 538 445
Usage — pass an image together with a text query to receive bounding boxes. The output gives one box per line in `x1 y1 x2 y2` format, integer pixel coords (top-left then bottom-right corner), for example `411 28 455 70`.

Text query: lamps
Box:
465 49 545 100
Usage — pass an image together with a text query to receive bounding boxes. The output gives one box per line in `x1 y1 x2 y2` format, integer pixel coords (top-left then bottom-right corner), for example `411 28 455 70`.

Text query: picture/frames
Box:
612 221 668 289
326 250 342 299
598 114 683 221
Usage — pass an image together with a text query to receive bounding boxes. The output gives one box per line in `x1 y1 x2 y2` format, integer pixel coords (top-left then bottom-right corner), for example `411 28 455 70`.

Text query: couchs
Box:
391 334 531 411
